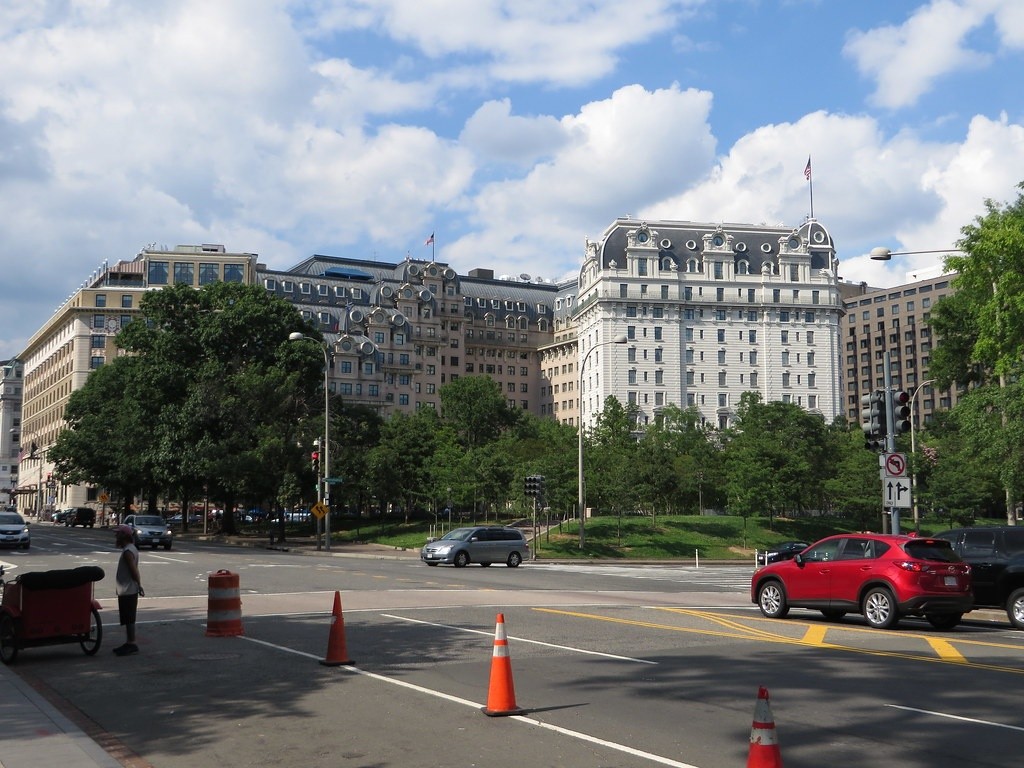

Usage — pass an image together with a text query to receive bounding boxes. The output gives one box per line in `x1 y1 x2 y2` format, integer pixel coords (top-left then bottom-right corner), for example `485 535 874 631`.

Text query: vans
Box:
64 507 96 530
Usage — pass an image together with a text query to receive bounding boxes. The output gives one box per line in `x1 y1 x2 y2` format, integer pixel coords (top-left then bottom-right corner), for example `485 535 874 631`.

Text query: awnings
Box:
9 485 38 495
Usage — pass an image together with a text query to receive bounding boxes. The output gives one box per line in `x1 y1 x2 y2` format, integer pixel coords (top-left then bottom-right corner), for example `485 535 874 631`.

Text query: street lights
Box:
577 337 629 550
289 332 331 550
868 248 1015 527
10 428 42 523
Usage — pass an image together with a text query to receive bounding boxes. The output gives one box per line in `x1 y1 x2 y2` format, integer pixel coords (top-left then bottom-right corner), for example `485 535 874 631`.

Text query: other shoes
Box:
113 643 139 657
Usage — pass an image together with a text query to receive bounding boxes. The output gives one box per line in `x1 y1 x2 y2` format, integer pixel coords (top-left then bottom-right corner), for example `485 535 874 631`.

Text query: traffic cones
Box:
316 590 356 668
480 613 528 717
744 685 785 767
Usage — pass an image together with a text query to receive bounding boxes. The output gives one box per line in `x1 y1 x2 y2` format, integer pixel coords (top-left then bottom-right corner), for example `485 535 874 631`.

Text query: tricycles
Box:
0 563 104 664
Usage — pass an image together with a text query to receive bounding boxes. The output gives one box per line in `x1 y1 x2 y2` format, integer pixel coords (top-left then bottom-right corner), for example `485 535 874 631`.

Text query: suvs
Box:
751 533 977 630
117 514 173 550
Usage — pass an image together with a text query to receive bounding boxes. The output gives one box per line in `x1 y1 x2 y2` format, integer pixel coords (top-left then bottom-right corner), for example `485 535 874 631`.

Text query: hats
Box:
112 525 133 537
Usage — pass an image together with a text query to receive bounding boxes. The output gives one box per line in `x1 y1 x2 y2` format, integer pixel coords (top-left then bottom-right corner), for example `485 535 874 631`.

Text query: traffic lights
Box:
524 475 546 498
890 389 912 437
861 390 884 437
863 431 884 452
312 451 321 474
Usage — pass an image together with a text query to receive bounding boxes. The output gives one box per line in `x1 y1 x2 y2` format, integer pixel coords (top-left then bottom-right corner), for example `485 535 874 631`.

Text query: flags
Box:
804 160 811 179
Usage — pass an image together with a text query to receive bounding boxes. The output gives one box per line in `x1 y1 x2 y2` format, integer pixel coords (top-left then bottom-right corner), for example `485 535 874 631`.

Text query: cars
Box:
755 542 811 565
421 525 530 568
927 523 1024 630
166 509 311 524
0 511 31 550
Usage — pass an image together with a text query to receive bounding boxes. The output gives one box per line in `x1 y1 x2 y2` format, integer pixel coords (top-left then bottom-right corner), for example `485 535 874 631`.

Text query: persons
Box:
10 497 14 507
51 511 61 526
111 525 145 657
98 510 103 525
